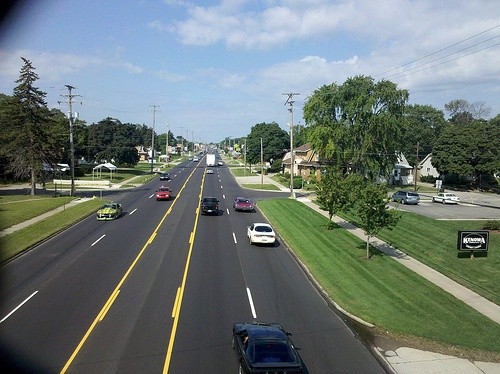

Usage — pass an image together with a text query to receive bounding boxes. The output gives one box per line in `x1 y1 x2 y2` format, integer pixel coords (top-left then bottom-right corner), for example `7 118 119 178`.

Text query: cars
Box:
193 158 199 162
247 223 276 245
160 173 170 180
155 187 172 200
232 321 309 374
233 196 254 212
432 193 460 205
206 167 214 174
218 160 224 165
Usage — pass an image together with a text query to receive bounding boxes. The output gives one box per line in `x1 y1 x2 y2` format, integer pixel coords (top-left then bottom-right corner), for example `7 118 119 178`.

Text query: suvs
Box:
200 197 219 215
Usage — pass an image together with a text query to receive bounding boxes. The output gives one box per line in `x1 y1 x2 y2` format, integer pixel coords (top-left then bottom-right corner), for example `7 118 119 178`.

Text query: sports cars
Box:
96 203 123 220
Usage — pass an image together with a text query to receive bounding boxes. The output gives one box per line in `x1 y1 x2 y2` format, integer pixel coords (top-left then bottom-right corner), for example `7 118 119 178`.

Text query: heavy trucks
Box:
206 154 216 166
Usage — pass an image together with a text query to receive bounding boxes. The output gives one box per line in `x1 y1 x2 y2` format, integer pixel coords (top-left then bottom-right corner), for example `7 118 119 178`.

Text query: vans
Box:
392 189 420 205
